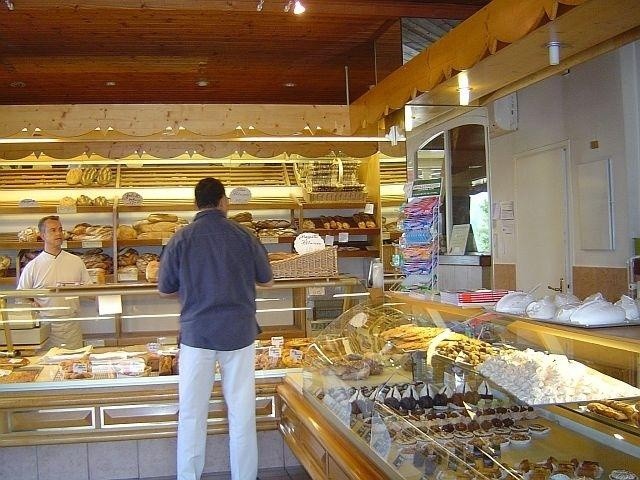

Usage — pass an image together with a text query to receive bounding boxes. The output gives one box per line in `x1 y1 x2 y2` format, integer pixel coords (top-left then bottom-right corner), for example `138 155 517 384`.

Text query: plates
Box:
394 422 551 461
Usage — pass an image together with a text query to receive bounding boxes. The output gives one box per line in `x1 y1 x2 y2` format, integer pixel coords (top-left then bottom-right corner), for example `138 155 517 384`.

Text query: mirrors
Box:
413 122 492 255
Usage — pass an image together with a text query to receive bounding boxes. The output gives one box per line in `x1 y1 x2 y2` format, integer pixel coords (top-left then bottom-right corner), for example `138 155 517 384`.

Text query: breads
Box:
0 166 376 282
0 324 316 384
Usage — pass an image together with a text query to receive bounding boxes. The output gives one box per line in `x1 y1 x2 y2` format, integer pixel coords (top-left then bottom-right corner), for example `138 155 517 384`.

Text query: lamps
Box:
543 42 562 65
456 87 473 106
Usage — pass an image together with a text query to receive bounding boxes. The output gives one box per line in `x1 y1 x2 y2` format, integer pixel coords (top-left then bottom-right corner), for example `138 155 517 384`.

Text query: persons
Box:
156 178 274 480
15 216 94 350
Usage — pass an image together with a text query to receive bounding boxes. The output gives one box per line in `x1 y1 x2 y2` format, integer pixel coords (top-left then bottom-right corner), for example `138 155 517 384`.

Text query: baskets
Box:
302 187 368 204
389 197 438 293
270 245 338 279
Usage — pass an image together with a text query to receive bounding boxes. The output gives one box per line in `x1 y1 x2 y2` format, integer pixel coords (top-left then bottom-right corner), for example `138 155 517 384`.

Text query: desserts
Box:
315 340 640 480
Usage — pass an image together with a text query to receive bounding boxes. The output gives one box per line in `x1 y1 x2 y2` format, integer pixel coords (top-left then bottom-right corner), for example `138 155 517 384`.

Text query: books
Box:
408 286 523 310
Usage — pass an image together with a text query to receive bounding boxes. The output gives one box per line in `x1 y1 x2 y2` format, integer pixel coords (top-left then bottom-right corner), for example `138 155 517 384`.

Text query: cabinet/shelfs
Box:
0 275 379 449
275 292 640 480
388 194 441 292
0 156 407 290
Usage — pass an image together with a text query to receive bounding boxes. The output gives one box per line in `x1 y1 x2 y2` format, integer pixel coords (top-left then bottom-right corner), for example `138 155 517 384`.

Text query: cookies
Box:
380 324 446 350
